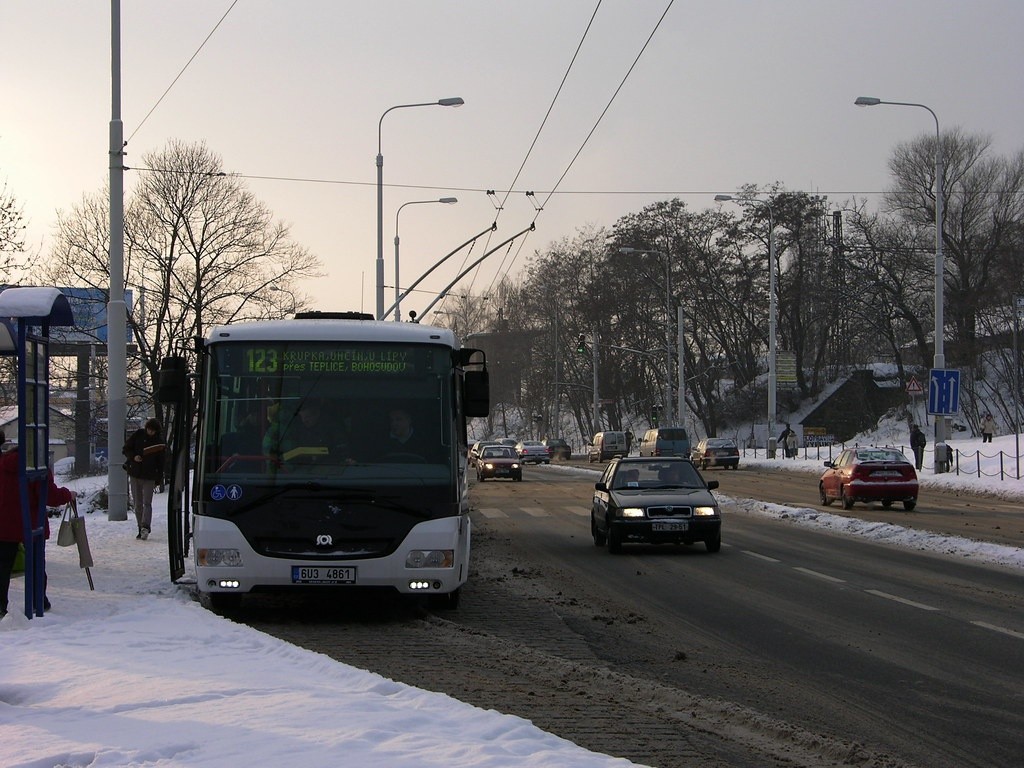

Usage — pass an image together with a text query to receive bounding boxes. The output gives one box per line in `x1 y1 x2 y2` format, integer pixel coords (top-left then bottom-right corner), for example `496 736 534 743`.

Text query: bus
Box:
157 312 491 612
90 414 198 472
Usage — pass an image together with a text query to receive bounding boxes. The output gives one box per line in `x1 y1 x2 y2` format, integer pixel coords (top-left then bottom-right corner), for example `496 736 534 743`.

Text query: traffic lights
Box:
577 331 586 354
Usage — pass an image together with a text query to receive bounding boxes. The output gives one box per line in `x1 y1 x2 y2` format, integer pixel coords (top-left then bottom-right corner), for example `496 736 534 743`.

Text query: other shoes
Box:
135 530 141 539
140 528 148 540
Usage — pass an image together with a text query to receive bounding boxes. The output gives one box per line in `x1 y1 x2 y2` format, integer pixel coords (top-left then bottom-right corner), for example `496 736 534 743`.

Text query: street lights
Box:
619 247 673 426
376 95 465 319
714 194 777 459
853 95 948 474
395 197 459 322
270 286 296 315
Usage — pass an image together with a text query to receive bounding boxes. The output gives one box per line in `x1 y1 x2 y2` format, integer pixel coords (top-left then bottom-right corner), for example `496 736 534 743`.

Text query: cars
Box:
475 444 525 483
512 440 551 465
818 442 919 511
541 438 571 460
591 454 722 556
691 437 740 471
467 437 518 468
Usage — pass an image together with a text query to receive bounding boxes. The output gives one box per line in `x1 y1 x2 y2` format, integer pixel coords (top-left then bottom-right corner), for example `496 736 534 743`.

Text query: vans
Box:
638 427 692 459
588 431 629 464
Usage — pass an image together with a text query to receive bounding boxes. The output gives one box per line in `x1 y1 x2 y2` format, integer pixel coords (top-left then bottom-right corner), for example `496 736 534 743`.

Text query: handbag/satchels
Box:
71 499 94 567
57 503 75 547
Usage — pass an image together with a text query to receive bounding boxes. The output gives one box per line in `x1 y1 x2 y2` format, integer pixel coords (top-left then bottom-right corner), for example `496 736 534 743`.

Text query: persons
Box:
911 426 926 469
946 445 953 472
346 406 430 466
624 428 633 453
0 429 77 618
777 424 798 457
665 464 688 484
238 396 342 475
123 418 168 541
980 415 996 442
